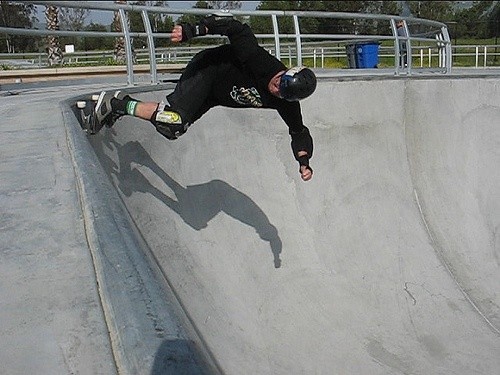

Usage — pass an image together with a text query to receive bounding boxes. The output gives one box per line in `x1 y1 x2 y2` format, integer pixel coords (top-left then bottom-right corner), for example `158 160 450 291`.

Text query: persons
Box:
90 19 317 183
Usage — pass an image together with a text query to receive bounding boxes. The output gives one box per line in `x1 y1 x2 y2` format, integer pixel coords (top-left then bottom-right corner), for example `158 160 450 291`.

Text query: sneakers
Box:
105 90 129 129
89 91 114 135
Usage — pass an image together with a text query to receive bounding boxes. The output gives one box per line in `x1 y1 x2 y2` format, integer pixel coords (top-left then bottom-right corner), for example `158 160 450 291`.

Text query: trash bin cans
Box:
355 40 383 68
344 43 357 69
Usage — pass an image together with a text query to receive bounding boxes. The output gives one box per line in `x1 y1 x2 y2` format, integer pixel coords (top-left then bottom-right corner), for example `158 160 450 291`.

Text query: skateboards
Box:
77 95 105 136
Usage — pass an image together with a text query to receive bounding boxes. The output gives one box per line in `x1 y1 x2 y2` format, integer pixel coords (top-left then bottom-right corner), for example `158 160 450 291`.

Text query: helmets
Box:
279 66 317 100
